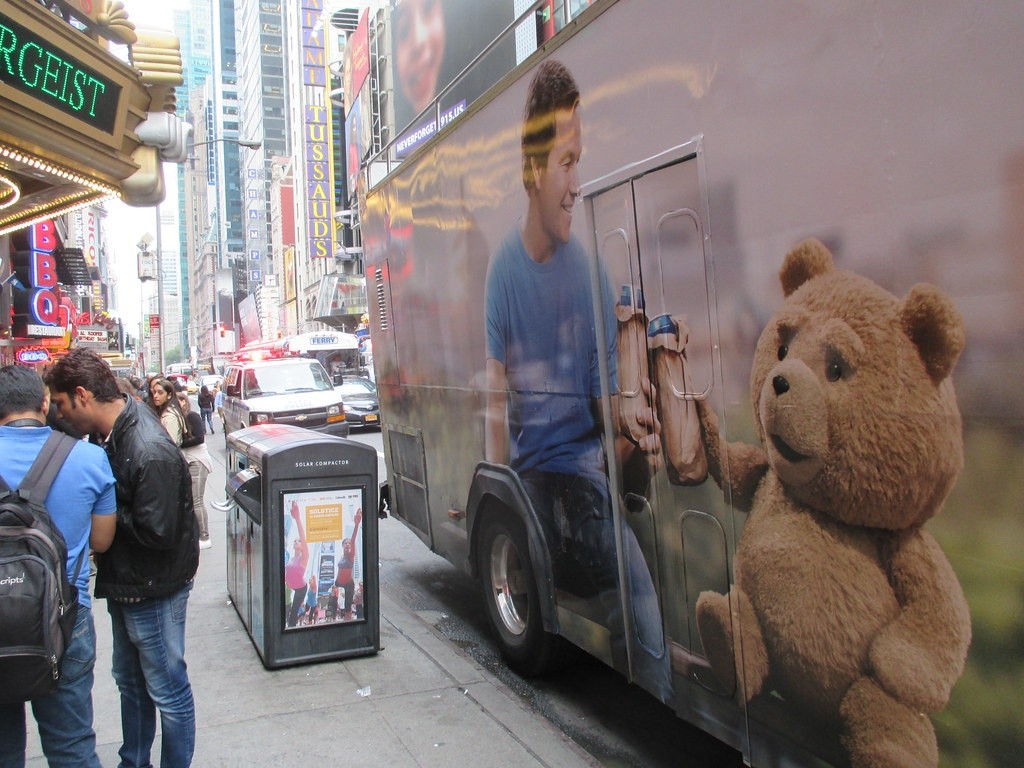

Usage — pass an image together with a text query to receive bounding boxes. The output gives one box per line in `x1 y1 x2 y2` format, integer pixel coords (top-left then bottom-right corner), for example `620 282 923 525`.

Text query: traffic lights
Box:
220 324 225 336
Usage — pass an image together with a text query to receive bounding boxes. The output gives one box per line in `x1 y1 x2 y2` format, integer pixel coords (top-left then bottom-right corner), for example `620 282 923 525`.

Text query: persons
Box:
43 347 202 768
285 500 364 628
331 354 346 374
42 371 213 549
215 385 226 430
481 60 674 704
0 364 117 768
197 385 216 436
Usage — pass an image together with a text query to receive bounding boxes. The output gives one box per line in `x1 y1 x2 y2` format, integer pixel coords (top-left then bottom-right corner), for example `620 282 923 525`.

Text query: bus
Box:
167 363 194 375
342 0 1024 768
236 330 360 380
196 356 236 377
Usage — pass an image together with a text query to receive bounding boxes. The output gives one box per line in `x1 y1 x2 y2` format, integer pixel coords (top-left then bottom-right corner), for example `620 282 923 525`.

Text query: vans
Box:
221 359 348 443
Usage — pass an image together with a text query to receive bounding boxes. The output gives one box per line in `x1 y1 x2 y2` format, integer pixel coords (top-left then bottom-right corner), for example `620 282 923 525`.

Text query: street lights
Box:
141 291 177 376
154 138 262 377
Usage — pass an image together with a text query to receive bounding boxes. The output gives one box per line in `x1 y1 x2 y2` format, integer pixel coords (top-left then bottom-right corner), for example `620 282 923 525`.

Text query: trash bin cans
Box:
225 422 383 668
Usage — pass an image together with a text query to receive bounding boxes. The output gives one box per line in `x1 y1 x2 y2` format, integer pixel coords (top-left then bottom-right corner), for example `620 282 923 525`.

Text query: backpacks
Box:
0 430 85 703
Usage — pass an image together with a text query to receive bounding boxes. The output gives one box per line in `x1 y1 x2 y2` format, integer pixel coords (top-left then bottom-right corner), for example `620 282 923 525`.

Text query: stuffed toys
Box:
697 236 972 767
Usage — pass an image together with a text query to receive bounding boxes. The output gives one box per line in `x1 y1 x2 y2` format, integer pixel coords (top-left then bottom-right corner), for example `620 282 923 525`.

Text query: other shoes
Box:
211 428 214 434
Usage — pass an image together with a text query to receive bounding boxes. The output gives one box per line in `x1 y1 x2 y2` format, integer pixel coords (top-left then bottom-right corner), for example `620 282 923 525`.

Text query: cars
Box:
198 375 225 393
330 376 383 432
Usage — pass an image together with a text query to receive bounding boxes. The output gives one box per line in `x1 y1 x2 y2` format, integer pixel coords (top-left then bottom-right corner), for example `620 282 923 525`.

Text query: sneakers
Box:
199 540 211 549
